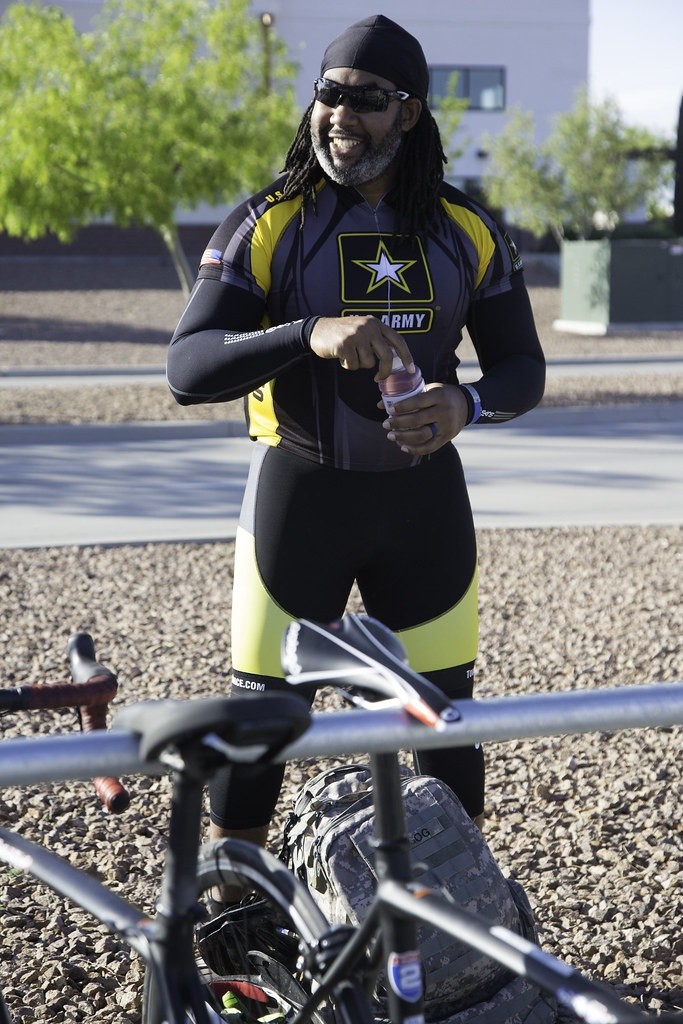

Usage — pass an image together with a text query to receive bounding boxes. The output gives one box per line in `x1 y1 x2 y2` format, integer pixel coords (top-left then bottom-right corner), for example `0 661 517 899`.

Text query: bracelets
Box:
463 382 481 427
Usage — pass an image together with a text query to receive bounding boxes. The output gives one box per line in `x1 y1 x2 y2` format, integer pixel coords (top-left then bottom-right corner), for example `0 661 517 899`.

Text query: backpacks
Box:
277 764 558 1024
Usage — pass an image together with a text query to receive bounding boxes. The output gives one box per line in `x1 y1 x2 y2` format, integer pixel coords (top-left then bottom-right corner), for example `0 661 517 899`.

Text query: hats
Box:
321 14 429 99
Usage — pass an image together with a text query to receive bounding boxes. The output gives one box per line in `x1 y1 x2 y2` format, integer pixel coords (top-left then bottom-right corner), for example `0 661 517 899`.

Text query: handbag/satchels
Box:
196 893 302 976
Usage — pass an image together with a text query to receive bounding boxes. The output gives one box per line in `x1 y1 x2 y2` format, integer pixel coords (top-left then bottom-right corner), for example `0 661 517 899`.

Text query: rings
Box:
427 423 438 440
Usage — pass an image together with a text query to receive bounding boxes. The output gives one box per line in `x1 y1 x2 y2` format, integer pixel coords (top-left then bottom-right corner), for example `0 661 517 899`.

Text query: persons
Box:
166 16 546 914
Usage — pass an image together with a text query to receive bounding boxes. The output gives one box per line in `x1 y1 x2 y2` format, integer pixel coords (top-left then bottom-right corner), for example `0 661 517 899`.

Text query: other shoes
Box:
203 887 253 918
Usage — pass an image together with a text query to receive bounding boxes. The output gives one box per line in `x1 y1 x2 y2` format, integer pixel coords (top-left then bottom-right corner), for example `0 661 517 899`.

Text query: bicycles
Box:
0 611 683 1024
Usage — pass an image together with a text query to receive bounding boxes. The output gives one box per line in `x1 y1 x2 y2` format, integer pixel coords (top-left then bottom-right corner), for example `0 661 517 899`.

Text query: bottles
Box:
378 349 426 446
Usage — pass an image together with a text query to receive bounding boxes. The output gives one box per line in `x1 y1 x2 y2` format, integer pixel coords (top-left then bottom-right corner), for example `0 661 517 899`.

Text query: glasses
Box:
314 78 407 115
